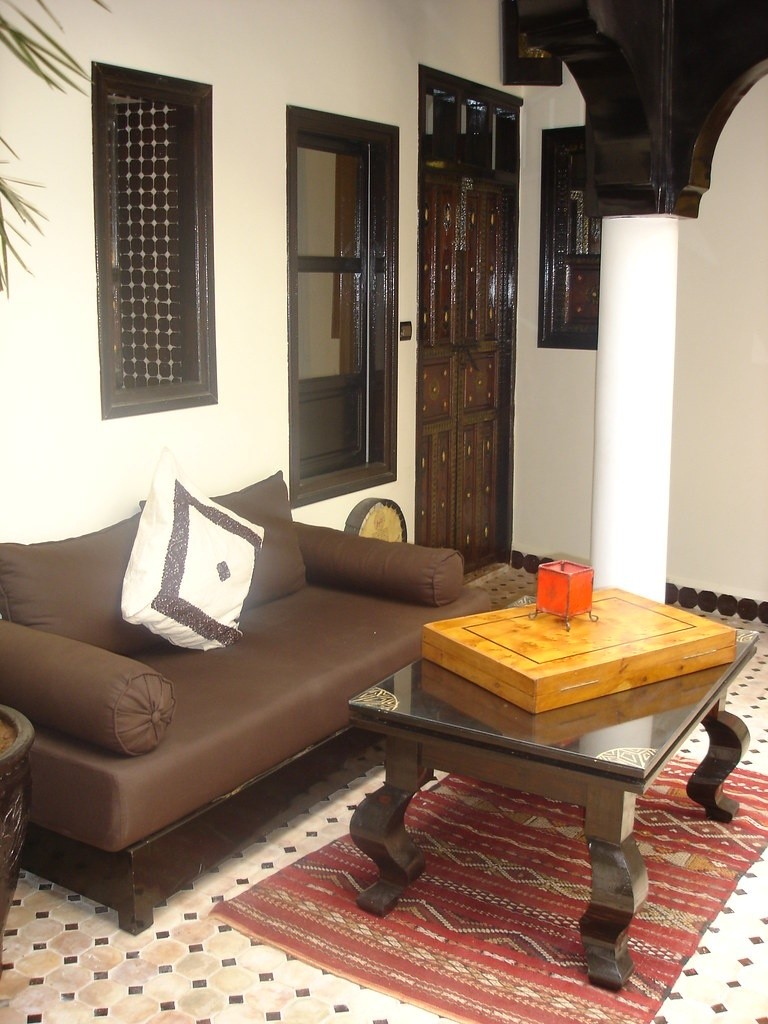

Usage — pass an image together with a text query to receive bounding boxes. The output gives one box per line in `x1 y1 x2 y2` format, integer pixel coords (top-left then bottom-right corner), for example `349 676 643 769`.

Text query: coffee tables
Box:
342 595 764 989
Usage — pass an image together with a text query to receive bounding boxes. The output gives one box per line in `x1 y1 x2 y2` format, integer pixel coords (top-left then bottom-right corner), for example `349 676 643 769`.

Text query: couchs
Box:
0 466 492 936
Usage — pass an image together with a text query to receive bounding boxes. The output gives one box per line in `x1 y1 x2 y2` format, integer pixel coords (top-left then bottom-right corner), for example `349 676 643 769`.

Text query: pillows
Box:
119 445 266 651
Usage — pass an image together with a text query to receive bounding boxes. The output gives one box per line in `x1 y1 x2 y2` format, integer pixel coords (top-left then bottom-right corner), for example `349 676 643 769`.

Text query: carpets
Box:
209 749 767 1024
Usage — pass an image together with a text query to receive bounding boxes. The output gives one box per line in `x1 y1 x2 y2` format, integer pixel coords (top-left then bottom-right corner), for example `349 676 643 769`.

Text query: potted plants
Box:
0 0 113 931
